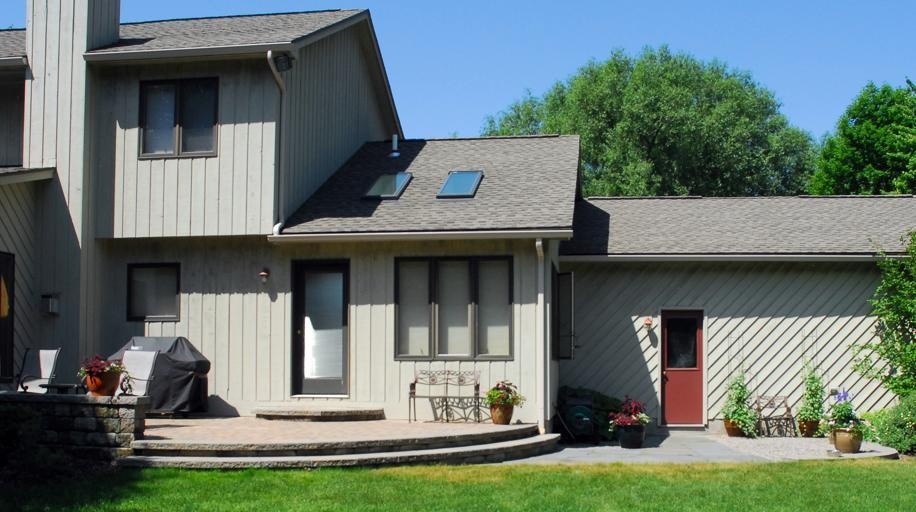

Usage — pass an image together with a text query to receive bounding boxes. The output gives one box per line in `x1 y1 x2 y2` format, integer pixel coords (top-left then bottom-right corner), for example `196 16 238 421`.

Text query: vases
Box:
490 404 514 423
85 372 120 397
615 423 647 448
828 427 863 452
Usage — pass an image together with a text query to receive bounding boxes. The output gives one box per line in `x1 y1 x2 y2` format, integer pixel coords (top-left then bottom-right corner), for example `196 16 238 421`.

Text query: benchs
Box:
409 370 481 422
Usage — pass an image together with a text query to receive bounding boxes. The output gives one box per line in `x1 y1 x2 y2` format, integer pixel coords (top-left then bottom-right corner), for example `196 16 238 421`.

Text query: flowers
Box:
827 389 882 444
75 354 134 393
607 395 653 432
481 379 528 408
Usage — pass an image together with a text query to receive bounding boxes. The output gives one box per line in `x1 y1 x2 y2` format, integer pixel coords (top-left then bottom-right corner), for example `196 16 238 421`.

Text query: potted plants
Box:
721 367 761 440
796 354 825 437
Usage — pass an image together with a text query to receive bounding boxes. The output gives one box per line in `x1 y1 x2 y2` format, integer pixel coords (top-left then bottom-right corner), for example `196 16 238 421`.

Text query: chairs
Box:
757 395 796 437
15 346 62 394
112 350 160 396
